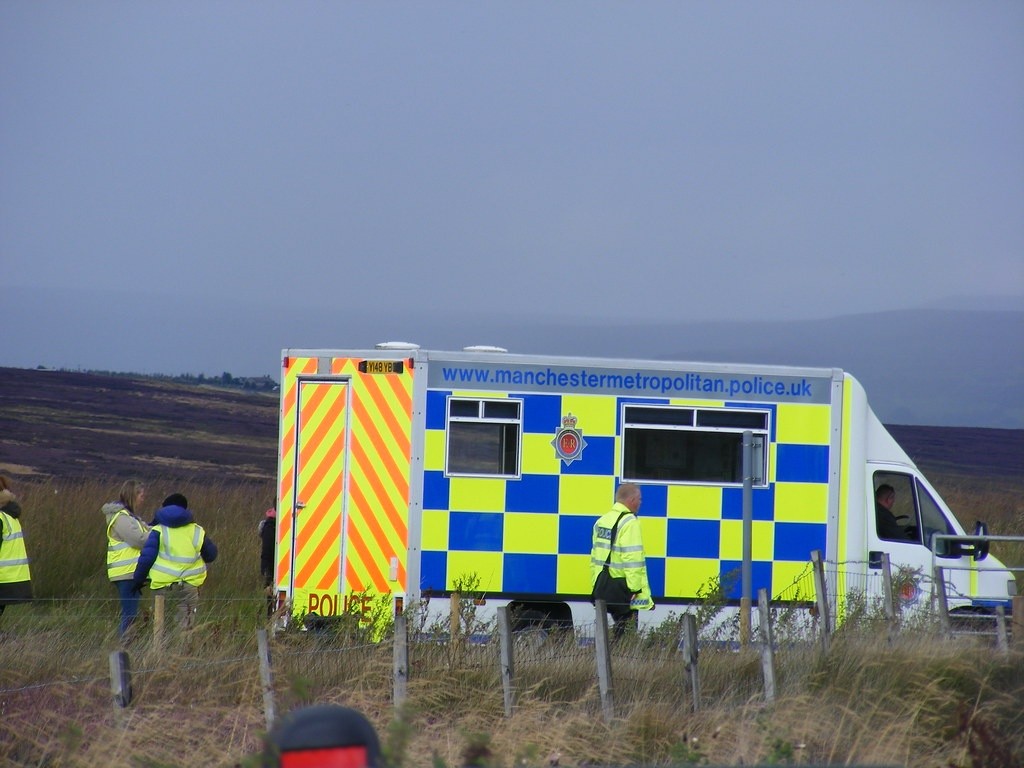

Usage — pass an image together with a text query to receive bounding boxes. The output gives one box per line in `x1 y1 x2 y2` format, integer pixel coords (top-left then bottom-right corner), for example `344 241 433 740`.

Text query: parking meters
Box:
260 704 382 768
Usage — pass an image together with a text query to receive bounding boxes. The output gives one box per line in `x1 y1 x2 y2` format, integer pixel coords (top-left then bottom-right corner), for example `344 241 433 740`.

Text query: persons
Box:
255 497 278 599
870 484 910 542
103 476 156 646
590 483 655 646
0 487 33 620
126 492 218 647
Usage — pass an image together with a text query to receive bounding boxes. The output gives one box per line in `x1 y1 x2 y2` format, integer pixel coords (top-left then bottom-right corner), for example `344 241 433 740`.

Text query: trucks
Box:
275 339 1015 671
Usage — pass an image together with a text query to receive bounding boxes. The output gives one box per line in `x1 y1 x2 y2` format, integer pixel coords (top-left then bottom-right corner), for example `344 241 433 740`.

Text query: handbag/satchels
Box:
590 570 635 619
